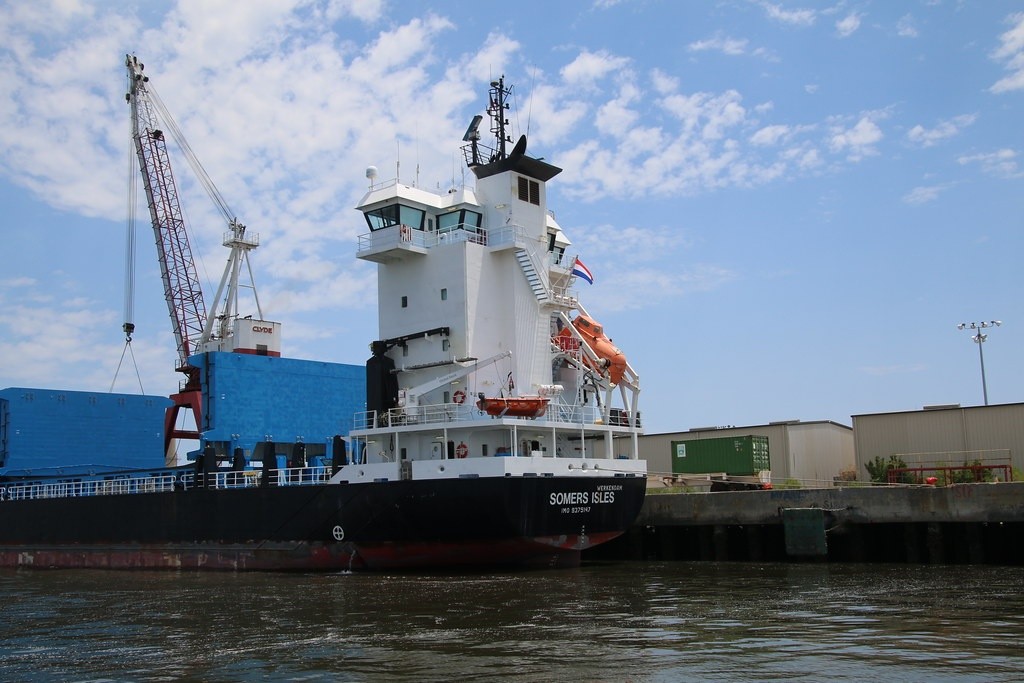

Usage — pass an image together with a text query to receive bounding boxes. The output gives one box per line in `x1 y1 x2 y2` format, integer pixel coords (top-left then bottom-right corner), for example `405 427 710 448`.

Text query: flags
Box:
572 259 594 285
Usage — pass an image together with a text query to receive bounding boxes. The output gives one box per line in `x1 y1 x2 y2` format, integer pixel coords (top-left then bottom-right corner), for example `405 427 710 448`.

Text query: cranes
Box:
124 52 287 460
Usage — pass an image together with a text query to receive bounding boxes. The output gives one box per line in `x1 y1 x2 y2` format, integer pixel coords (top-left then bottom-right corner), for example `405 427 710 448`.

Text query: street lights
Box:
958 320 1002 405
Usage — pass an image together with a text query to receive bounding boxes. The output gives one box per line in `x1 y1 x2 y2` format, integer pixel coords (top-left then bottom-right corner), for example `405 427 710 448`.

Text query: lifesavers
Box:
452 390 465 407
456 444 468 458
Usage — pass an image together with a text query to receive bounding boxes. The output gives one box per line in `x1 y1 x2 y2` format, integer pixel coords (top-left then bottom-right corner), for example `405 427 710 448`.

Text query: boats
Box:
1 72 650 572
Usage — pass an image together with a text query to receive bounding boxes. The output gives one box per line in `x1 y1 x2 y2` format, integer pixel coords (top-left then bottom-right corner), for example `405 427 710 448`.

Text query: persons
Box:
402 224 408 241
